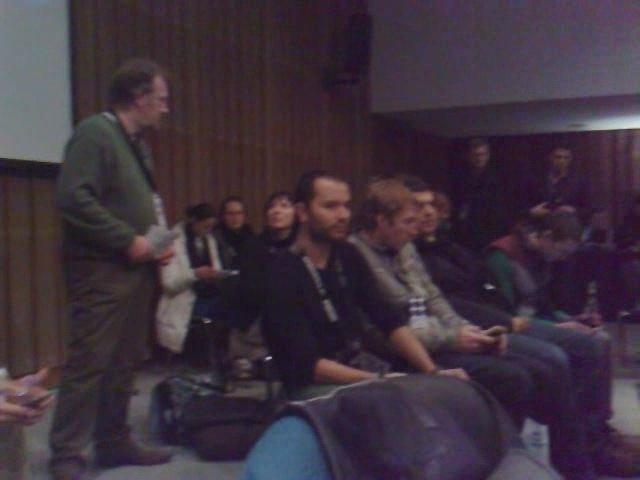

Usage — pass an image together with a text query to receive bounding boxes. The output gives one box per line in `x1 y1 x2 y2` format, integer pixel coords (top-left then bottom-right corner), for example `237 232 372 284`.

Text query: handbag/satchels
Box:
184 393 277 461
155 375 224 446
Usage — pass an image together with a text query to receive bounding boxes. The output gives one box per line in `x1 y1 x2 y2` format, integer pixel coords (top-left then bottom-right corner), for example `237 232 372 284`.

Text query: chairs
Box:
141 252 339 461
240 374 565 478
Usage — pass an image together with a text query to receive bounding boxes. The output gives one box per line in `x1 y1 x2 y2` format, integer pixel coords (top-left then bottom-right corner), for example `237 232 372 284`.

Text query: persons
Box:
44 55 180 480
0 362 54 480
155 134 639 479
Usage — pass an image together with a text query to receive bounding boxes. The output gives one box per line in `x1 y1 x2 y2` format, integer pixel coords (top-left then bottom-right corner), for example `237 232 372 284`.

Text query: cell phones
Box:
591 321 608 333
20 389 56 410
485 325 508 338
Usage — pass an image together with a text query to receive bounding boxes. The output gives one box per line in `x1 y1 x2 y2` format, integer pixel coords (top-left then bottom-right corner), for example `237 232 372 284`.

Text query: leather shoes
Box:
548 415 640 480
91 428 174 468
50 458 84 480
